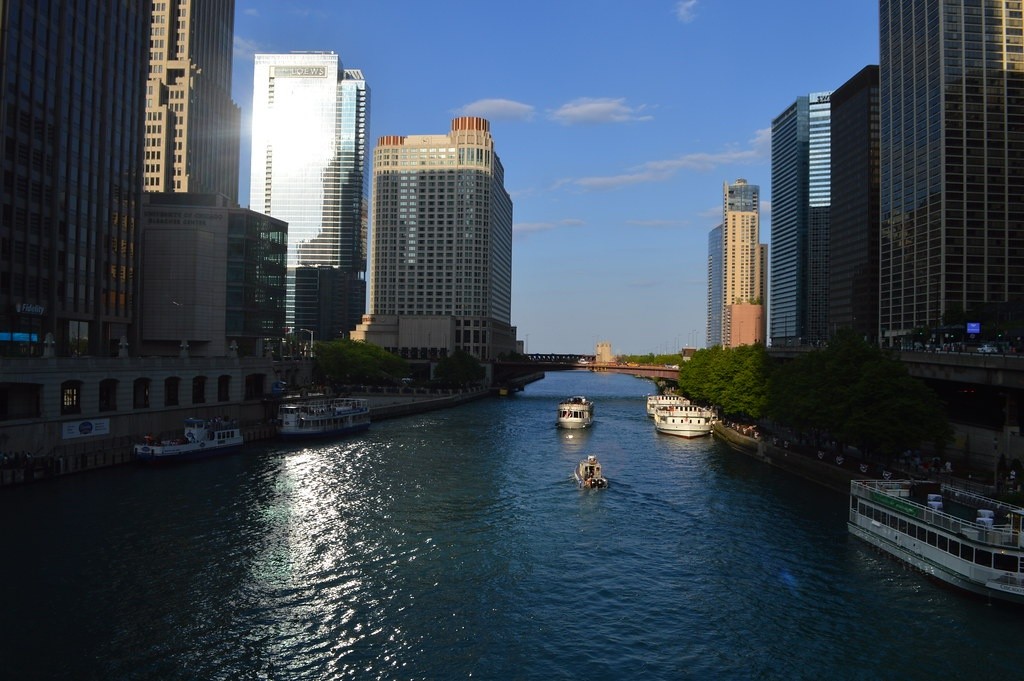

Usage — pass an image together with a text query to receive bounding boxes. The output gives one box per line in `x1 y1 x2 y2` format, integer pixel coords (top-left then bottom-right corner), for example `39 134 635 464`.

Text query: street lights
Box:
300 328 314 358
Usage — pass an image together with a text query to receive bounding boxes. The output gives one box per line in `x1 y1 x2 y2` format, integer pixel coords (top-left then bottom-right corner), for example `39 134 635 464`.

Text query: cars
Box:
977 344 998 354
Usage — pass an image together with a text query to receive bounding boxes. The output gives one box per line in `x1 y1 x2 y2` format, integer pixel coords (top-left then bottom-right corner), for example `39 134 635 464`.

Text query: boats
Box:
845 479 1024 607
134 419 245 460
647 394 691 418
574 455 608 491
654 404 714 440
276 397 371 441
554 396 595 430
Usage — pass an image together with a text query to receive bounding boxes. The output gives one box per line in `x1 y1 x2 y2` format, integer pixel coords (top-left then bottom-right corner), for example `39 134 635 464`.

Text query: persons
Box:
144 433 162 446
807 427 817 448
208 416 222 425
997 471 1018 496
0 451 32 467
1002 339 1016 354
178 436 189 444
822 429 834 453
584 467 600 481
904 448 953 477
